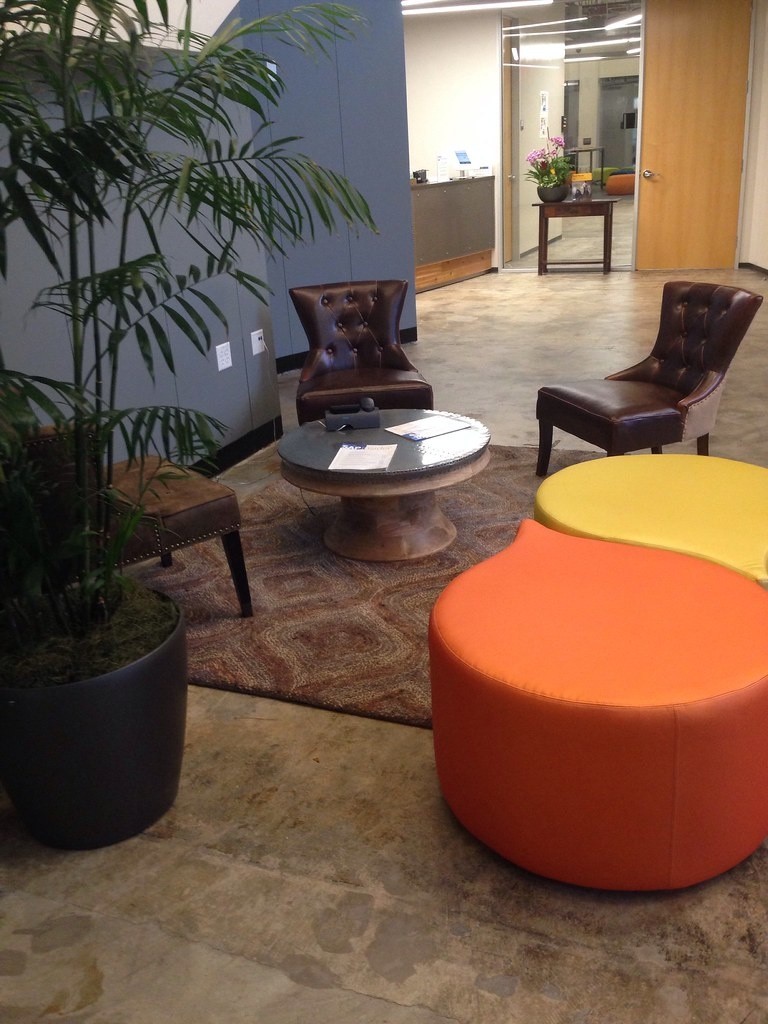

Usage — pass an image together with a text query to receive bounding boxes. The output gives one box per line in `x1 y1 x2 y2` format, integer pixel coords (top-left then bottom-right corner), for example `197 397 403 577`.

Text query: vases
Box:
537 183 569 202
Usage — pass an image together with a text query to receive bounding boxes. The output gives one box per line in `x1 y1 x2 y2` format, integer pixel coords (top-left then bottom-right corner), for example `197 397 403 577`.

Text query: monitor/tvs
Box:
623 113 636 129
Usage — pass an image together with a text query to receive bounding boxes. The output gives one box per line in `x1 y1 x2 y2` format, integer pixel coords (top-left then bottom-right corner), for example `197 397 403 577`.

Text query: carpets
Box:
131 443 610 733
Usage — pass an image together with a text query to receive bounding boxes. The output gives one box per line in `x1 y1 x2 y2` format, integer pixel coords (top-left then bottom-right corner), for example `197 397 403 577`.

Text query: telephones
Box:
324 395 382 433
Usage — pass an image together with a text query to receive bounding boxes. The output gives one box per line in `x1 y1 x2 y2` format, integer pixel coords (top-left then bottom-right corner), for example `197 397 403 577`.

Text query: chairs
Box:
23 424 254 617
533 281 764 478
288 278 433 422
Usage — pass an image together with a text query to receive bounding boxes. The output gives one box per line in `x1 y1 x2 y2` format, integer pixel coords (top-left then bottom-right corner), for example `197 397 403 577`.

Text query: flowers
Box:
524 136 577 189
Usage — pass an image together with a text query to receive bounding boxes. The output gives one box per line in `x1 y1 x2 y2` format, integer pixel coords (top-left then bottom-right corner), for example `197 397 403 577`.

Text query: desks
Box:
278 408 492 560
568 146 605 190
530 197 623 275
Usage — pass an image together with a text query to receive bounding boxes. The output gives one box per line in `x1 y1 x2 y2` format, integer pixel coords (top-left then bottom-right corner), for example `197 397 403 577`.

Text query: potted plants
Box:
0 0 381 851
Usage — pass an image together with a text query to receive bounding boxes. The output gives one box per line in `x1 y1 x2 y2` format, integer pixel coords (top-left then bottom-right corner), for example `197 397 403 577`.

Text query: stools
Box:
593 166 634 196
425 517 768 893
532 452 768 589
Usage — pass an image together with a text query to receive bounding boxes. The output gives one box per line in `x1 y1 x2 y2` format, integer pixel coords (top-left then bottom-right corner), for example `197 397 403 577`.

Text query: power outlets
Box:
215 341 232 371
251 329 265 356
